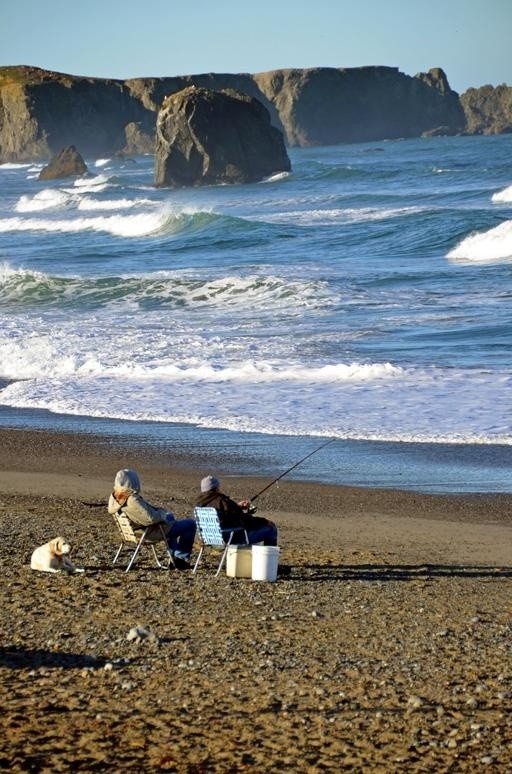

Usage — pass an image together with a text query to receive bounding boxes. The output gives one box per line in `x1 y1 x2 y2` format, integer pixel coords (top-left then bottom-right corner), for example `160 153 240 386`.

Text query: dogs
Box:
29 536 86 573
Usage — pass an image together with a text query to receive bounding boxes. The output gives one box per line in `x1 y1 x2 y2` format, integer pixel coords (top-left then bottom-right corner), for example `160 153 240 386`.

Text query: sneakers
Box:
169 558 192 570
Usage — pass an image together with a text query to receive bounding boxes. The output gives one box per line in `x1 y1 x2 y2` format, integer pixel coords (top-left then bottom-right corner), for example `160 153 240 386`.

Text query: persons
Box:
196 474 293 576
107 467 199 572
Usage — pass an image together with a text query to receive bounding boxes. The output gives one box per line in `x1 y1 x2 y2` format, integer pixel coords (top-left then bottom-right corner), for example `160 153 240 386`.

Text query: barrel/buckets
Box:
227 549 252 578
251 545 280 581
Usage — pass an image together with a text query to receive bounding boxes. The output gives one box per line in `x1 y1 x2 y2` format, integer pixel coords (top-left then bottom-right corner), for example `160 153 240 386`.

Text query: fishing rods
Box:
240 428 359 513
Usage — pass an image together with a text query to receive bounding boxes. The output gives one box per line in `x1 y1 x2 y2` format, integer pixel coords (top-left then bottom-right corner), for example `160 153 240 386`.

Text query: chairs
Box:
193 507 253 578
108 511 176 573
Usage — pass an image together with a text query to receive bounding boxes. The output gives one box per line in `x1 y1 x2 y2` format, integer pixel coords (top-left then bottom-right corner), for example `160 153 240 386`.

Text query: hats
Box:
201 475 219 493
